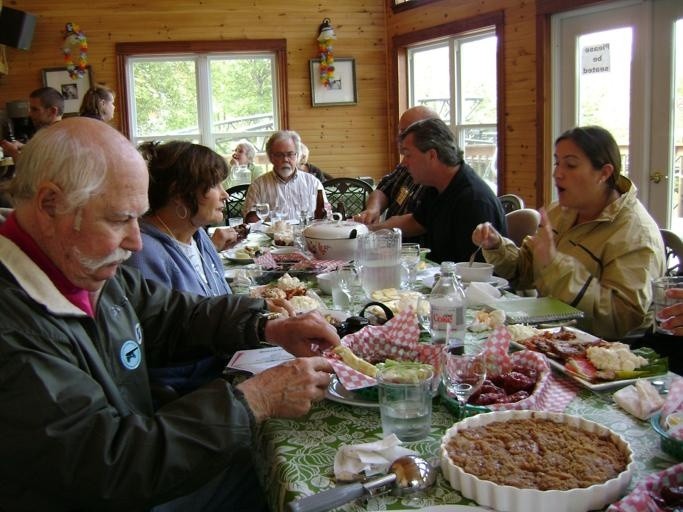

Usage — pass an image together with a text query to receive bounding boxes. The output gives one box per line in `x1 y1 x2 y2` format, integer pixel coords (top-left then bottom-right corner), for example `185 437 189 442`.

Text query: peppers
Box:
459 366 538 403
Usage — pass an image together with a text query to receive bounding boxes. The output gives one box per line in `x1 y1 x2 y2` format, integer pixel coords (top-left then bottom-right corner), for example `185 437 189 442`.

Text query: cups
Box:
229 218 244 227
332 269 356 310
376 364 434 441
649 277 683 336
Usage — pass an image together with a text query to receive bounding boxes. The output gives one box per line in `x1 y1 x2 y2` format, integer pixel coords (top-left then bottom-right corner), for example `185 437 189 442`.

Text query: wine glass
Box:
400 242 421 291
256 202 270 225
273 206 291 220
292 195 312 225
440 344 488 420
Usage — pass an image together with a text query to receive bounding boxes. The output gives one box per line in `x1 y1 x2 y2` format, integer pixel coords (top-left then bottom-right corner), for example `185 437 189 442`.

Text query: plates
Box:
438 410 635 510
323 358 384 407
221 245 261 263
510 326 667 391
271 237 294 248
415 266 509 292
224 268 263 280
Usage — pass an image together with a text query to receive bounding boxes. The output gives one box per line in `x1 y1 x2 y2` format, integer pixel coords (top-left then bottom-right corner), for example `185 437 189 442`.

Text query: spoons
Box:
287 455 436 511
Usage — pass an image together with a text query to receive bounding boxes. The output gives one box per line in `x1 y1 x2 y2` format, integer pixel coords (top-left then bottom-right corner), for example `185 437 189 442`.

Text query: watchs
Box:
257 312 281 343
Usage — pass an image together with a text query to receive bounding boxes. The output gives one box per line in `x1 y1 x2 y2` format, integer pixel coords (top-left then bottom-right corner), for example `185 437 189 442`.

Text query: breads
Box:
371 287 431 320
331 342 435 384
442 417 632 491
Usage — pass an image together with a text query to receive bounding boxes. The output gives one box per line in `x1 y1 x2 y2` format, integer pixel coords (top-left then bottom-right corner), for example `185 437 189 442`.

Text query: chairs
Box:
498 194 524 214
226 184 250 218
660 229 682 280
322 177 373 220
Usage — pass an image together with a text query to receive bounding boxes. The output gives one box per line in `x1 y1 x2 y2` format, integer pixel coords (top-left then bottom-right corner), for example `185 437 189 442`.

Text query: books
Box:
484 294 584 325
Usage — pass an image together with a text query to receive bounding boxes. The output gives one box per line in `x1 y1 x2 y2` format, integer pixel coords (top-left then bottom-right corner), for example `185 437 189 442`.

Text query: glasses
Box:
272 150 297 161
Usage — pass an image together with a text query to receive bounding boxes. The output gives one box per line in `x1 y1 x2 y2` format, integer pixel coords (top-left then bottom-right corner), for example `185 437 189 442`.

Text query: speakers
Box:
0 5 37 51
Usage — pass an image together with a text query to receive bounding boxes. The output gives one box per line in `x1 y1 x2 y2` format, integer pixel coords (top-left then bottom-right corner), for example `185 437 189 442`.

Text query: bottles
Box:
429 261 465 342
313 189 327 223
334 203 346 220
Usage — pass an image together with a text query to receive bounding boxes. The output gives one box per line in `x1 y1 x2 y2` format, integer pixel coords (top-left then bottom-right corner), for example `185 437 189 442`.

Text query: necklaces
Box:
155 212 221 298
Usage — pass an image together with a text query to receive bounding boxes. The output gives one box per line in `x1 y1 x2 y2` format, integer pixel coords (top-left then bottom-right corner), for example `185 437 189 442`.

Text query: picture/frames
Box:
41 64 94 118
310 57 358 107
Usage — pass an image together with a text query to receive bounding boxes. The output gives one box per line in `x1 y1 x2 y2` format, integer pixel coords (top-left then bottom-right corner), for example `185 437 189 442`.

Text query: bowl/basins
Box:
454 262 494 282
316 273 332 295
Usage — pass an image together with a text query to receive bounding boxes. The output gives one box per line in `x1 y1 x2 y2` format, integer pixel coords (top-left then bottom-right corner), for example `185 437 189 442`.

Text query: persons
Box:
80 84 116 123
0 117 340 511
381 116 508 265
471 126 667 341
223 140 256 210
619 287 683 377
354 105 440 243
211 228 241 252
296 143 326 184
243 130 334 221
0 87 65 159
122 140 297 398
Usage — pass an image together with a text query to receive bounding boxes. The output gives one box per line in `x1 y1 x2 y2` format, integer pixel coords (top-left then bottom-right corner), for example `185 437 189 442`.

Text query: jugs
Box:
354 228 400 300
